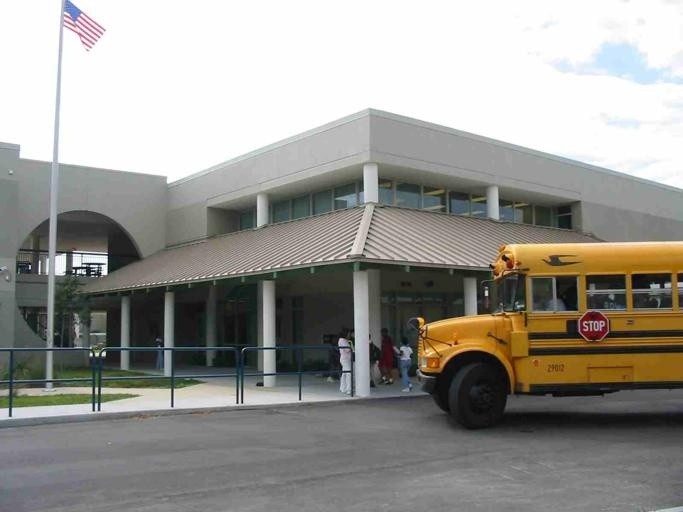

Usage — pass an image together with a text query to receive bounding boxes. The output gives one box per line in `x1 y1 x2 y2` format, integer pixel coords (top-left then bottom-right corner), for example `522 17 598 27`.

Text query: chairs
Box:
647 298 658 308
562 286 576 310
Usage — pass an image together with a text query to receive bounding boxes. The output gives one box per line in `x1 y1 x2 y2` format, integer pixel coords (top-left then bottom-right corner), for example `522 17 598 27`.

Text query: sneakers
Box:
340 375 412 395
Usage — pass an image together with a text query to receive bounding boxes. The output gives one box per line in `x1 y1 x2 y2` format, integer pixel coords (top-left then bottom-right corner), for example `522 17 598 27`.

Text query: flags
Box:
60 1 106 53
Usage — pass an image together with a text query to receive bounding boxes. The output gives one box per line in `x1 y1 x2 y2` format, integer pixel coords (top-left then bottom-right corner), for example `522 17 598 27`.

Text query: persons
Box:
325 328 377 395
392 335 414 393
377 327 394 385
580 280 683 308
537 281 568 313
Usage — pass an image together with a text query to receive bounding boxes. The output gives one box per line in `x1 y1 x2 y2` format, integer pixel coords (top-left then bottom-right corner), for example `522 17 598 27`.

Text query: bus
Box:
403 240 683 429
